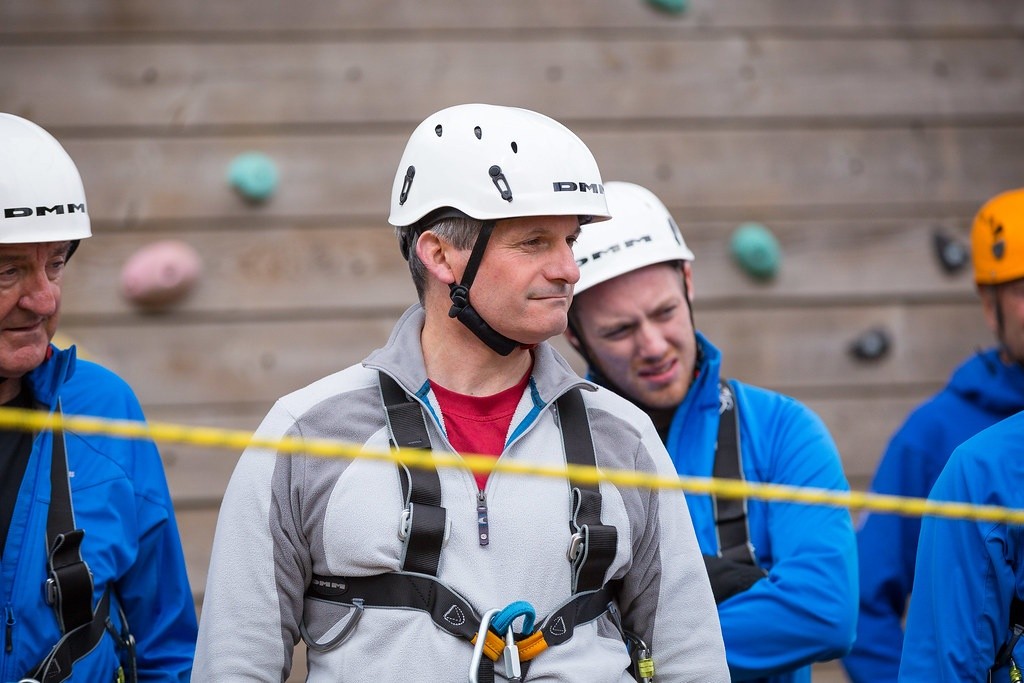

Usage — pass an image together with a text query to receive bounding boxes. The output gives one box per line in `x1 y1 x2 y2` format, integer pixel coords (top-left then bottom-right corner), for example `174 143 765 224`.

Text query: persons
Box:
190 104 731 683
845 188 1024 683
0 112 199 683
564 181 859 683
896 409 1024 683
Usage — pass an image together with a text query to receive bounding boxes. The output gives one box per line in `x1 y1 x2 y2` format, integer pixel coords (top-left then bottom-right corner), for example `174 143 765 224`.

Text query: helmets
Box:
387 103 611 232
572 180 696 297
970 188 1024 282
0 111 92 243
